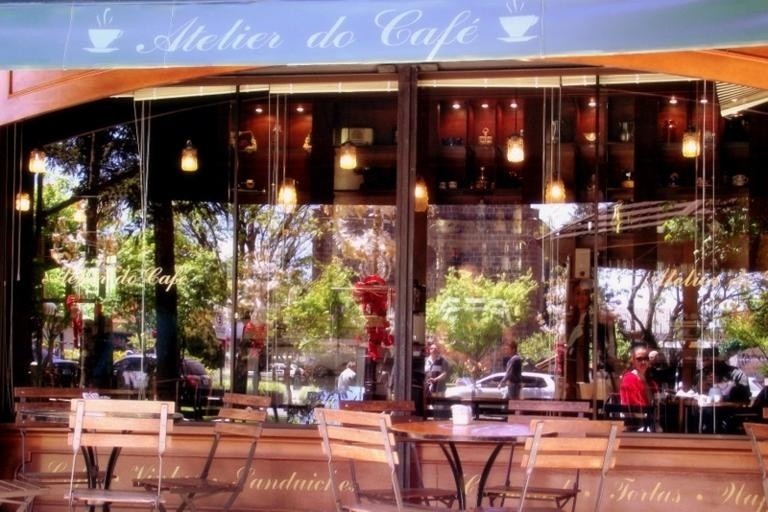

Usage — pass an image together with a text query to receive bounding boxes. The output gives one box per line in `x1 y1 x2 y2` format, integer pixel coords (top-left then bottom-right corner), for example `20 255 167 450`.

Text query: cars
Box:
30 335 615 426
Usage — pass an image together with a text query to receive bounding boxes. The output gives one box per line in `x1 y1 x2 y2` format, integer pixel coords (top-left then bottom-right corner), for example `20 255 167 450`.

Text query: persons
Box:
498 341 522 399
692 349 750 433
338 359 356 389
425 343 450 396
566 278 604 360
619 345 660 432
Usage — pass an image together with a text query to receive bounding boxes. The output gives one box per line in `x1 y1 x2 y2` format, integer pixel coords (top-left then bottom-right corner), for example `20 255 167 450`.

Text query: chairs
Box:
1 386 272 511
743 422 768 504
